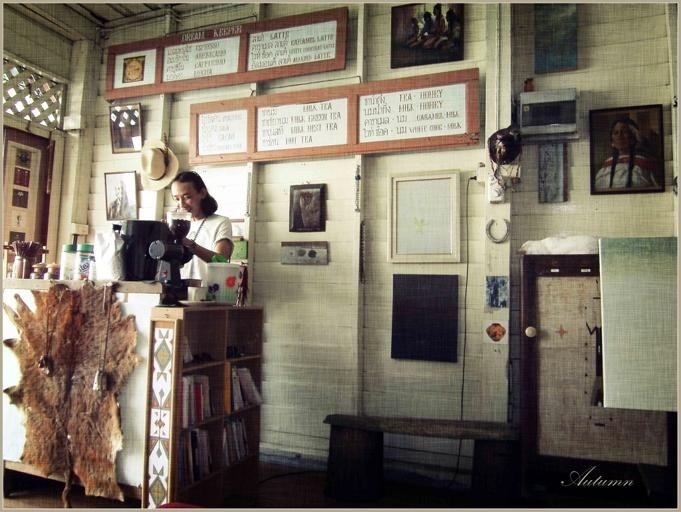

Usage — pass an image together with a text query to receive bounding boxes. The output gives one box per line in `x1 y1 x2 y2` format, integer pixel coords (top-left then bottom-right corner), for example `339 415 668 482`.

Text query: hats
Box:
139 140 180 192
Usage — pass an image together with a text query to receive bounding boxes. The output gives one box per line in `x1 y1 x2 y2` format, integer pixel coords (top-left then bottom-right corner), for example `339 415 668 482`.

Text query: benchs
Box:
324 412 513 508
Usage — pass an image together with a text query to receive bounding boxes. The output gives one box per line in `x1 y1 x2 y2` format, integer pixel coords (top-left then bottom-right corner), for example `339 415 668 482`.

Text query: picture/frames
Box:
109 103 145 155
103 170 139 222
385 168 460 263
588 104 663 193
190 68 480 164
288 184 326 233
389 4 463 69
105 7 347 103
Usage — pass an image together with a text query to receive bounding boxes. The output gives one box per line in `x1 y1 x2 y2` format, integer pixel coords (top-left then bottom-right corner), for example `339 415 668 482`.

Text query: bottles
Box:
60 243 76 281
74 244 96 281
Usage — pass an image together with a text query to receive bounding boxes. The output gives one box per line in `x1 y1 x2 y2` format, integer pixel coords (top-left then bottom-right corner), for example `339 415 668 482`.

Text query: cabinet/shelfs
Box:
140 302 262 508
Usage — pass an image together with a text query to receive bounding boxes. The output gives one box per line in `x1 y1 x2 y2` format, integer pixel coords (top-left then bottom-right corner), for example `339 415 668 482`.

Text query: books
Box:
179 366 262 489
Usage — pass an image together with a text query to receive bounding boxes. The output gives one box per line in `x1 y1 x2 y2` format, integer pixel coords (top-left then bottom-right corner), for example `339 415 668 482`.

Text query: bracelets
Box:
187 239 197 252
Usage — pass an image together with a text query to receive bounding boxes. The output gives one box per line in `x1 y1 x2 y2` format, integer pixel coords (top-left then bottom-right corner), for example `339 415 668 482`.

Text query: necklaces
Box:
185 216 206 241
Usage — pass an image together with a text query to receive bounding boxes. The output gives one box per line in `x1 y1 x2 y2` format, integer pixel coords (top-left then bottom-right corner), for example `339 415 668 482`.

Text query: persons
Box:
114 111 135 148
403 2 460 61
594 117 658 191
495 133 517 164
159 170 235 288
